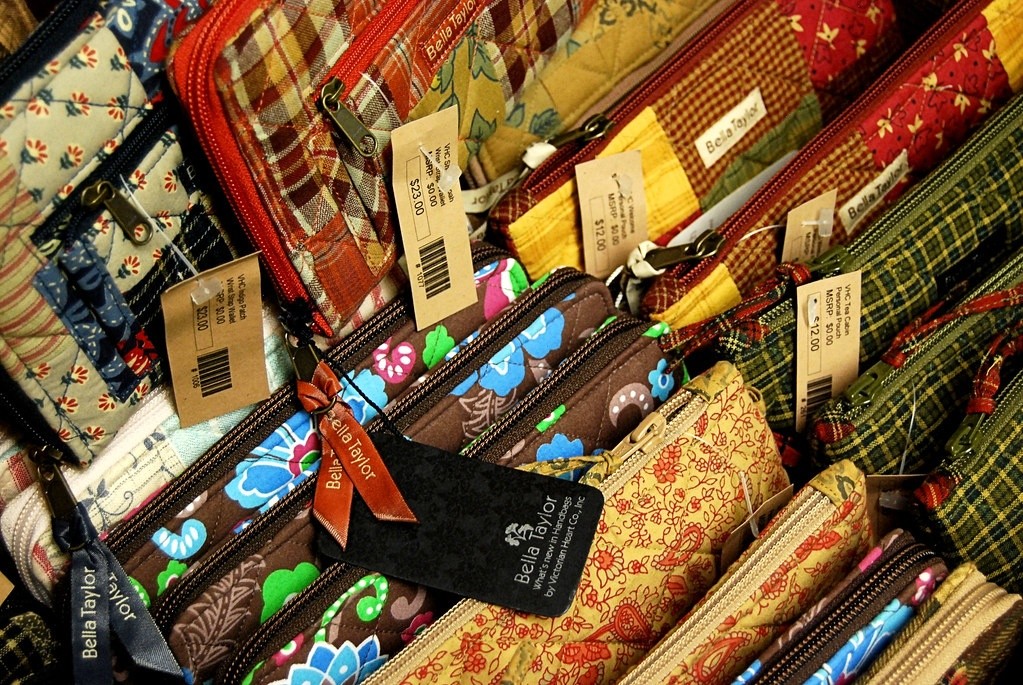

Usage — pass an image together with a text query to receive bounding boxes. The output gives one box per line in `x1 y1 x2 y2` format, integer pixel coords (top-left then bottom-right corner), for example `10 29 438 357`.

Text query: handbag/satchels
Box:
0 0 1023 685
